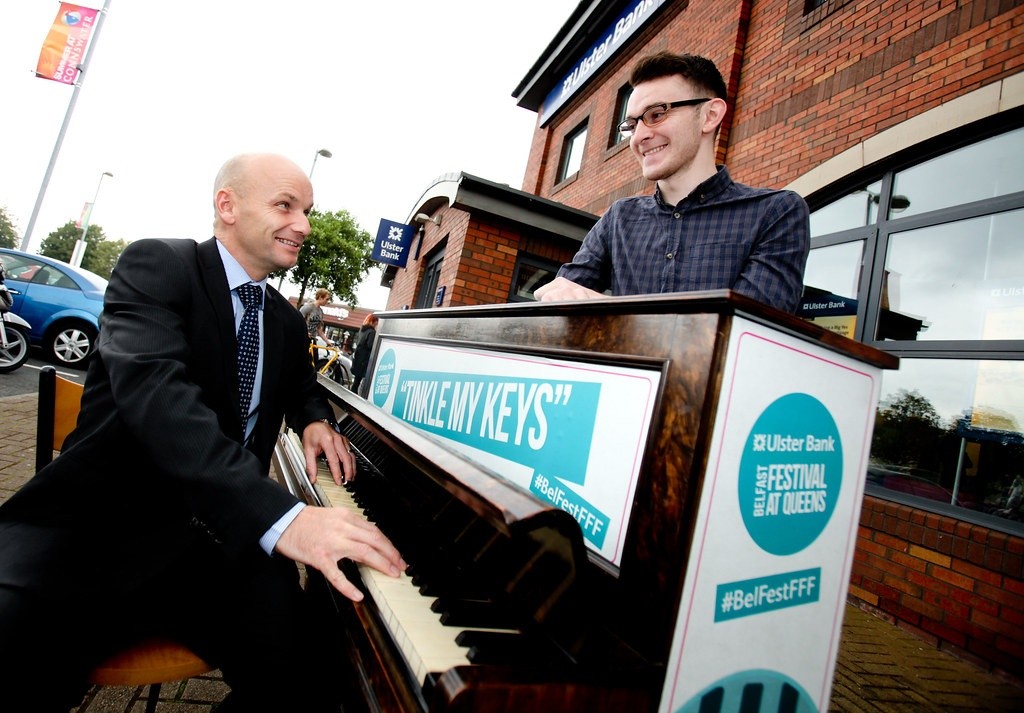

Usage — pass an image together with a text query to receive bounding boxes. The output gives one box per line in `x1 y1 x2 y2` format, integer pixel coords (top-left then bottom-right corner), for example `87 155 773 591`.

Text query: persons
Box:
299 288 340 347
534 51 810 315
351 315 378 395
0 152 407 713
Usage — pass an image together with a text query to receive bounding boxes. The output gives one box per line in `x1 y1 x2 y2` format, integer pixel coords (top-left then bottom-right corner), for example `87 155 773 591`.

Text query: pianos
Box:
271 289 900 713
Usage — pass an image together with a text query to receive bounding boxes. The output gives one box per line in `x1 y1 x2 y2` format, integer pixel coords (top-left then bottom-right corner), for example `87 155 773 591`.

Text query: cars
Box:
0 247 110 369
311 335 355 385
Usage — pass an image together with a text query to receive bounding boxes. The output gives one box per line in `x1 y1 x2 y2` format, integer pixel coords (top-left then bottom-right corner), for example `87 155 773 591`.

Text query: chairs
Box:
35 365 219 713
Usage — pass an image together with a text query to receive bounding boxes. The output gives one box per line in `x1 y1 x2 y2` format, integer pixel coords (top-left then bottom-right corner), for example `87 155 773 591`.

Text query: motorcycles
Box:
1 260 33 374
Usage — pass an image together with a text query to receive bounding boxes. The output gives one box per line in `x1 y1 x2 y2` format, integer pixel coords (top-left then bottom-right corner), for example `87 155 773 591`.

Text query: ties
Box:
236 283 263 437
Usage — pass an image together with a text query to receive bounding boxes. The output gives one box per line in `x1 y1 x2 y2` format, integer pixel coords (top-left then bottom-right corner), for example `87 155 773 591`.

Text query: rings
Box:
347 450 353 453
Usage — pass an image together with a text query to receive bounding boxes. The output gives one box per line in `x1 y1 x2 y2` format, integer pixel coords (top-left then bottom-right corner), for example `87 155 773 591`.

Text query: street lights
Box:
309 149 332 180
73 171 114 266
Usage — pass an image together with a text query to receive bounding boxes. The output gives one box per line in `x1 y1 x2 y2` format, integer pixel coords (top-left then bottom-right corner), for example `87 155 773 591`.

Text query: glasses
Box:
616 97 712 138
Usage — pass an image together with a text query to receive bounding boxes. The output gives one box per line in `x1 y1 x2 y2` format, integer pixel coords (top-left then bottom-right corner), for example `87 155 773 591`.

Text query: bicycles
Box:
306 334 351 391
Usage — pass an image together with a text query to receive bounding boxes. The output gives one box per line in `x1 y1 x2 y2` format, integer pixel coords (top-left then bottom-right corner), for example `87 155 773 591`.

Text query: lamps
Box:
415 212 442 226
